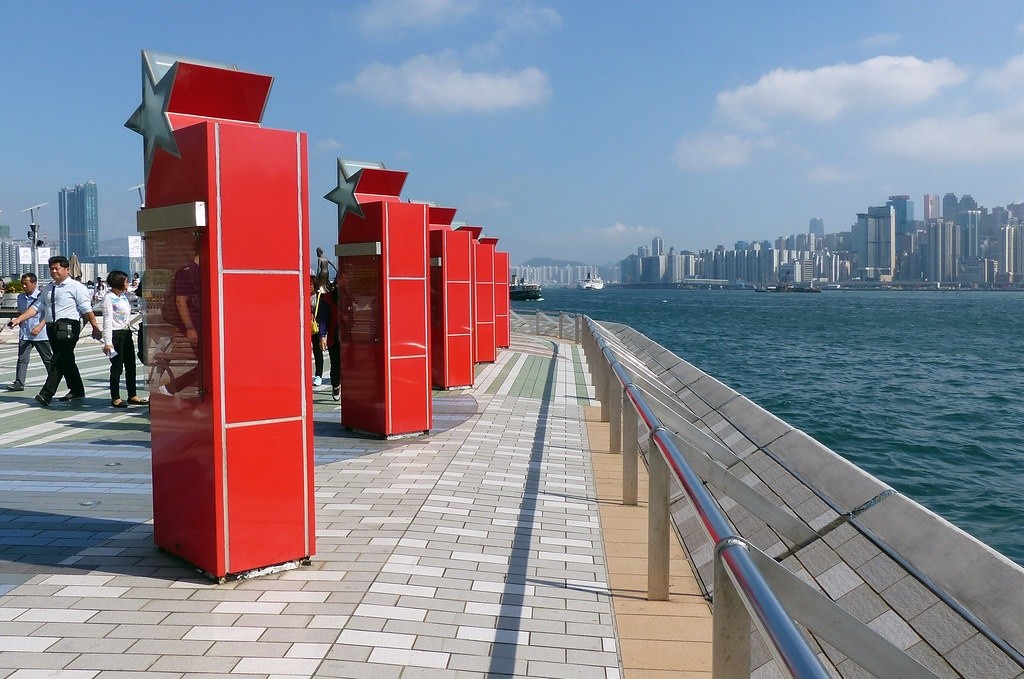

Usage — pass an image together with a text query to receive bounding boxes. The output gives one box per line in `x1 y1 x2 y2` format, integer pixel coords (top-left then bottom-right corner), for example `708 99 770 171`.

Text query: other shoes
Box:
111 399 128 407
127 396 149 404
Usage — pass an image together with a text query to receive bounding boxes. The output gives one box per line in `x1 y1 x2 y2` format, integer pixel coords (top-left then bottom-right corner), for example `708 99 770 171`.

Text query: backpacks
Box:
160 266 196 326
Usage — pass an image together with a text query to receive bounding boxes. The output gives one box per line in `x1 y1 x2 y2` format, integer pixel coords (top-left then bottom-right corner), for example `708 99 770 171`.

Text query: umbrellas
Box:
69 254 82 277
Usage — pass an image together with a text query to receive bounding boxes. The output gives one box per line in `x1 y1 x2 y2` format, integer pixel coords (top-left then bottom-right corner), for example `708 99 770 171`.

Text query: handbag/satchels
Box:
52 318 80 343
311 318 319 335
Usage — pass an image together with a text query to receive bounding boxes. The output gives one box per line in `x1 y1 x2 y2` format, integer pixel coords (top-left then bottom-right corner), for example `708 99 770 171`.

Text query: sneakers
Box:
193 409 208 419
332 384 341 400
6 379 24 391
312 375 322 385
159 385 173 397
59 393 86 403
35 395 51 405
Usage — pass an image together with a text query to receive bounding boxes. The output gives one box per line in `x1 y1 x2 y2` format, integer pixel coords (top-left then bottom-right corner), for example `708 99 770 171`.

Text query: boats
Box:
753 281 824 294
510 274 545 302
577 273 604 291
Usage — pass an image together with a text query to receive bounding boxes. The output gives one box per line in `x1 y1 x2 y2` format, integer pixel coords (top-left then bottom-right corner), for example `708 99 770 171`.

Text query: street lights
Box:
21 202 51 288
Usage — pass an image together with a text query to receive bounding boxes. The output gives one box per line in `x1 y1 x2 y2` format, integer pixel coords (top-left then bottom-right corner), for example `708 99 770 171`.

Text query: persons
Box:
132 272 140 288
6 273 52 390
94 278 108 302
159 240 201 397
103 271 146 408
8 256 102 406
310 275 341 401
318 247 338 293
